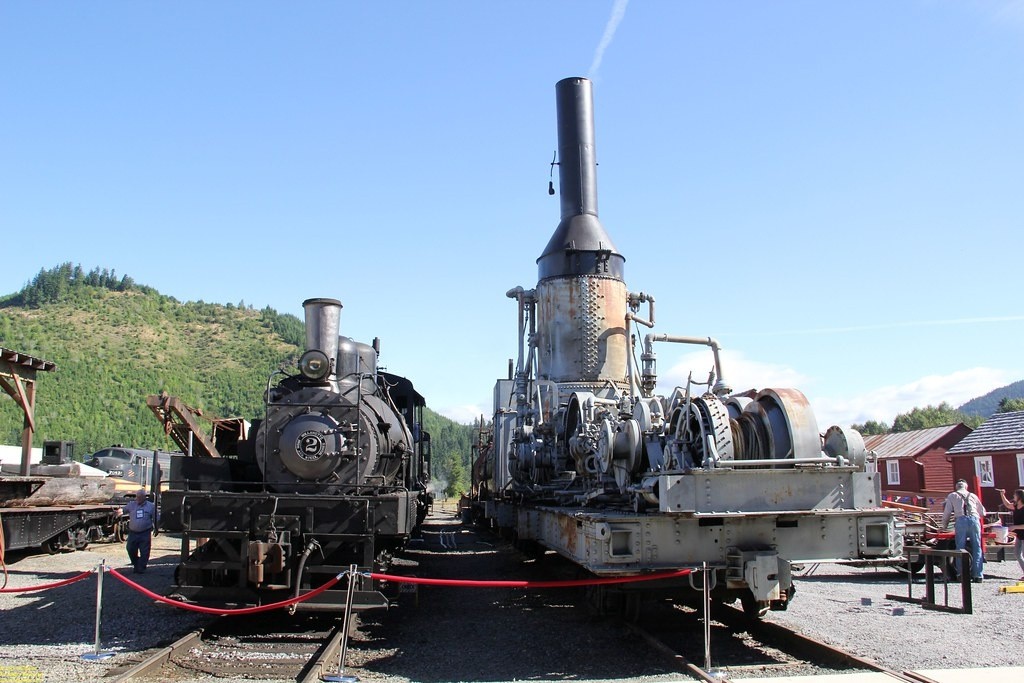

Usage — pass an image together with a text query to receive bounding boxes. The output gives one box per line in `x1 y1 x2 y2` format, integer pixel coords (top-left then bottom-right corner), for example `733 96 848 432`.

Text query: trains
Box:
0 345 176 560
142 296 439 622
446 74 908 635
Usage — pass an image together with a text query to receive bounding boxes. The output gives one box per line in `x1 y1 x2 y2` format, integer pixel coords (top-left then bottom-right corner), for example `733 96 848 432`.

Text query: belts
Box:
130 528 151 534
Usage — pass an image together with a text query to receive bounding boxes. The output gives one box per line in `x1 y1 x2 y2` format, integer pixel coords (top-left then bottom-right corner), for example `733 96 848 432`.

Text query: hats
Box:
956 479 966 483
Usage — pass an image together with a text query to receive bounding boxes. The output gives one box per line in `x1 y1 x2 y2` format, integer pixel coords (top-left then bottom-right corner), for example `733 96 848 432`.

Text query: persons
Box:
116 490 160 573
999 488 1024 581
939 479 986 582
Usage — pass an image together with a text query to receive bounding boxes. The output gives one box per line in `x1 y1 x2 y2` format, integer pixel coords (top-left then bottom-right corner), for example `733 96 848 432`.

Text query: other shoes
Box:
1019 577 1024 581
138 566 146 574
972 576 982 582
957 575 963 582
